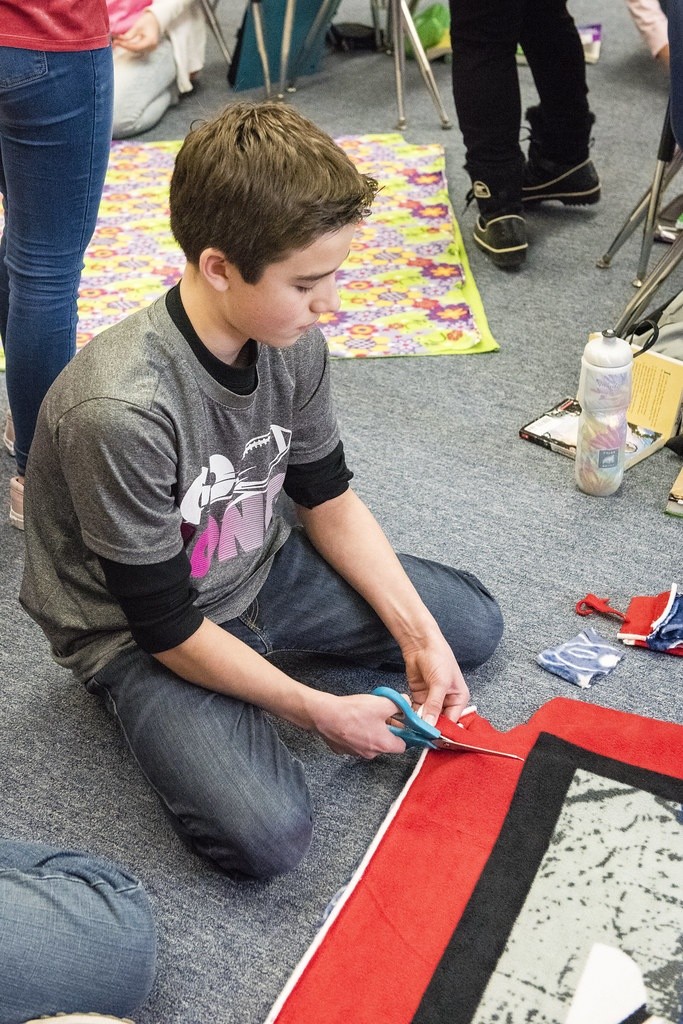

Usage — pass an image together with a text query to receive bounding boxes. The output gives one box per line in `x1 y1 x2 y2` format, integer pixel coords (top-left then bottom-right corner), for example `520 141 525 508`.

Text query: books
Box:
517 394 666 475
582 328 683 442
664 465 683 518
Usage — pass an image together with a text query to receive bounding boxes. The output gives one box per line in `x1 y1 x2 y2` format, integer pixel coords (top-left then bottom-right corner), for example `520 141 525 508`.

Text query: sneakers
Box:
2 411 17 455
526 160 601 206
9 475 26 529
470 215 530 265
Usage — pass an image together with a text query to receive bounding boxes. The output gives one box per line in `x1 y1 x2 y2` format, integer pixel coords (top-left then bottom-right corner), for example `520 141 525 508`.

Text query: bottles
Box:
574 330 633 496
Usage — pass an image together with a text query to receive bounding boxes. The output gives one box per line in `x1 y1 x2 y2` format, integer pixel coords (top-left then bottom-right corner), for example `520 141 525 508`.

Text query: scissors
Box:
369 687 524 761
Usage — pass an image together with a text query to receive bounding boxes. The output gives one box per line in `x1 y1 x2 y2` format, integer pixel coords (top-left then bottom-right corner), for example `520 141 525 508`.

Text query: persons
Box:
17 99 516 889
98 0 209 141
0 0 116 532
443 1 605 274
621 0 670 67
0 827 163 1024
